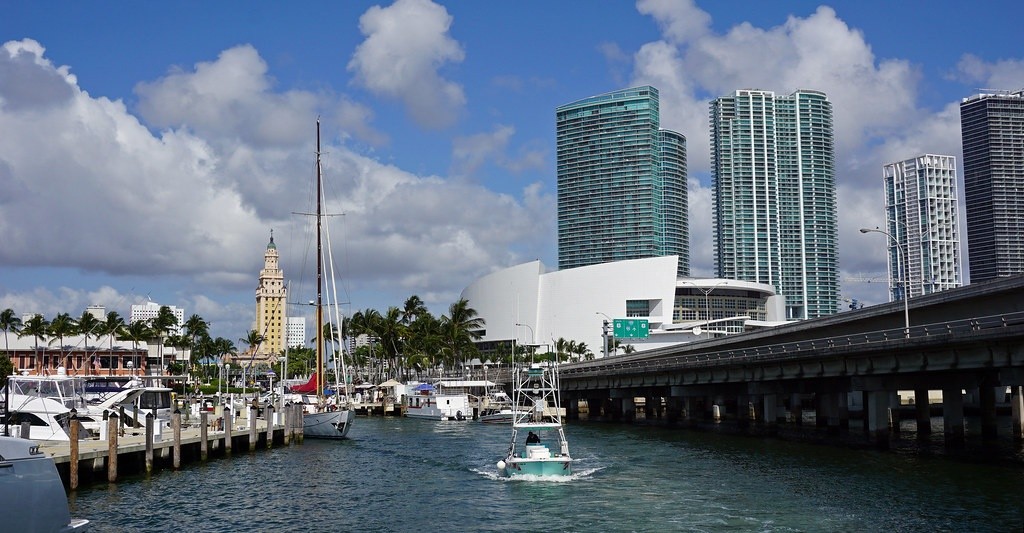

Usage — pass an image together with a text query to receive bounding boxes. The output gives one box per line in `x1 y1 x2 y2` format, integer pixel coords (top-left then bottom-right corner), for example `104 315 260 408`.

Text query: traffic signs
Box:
614 319 648 339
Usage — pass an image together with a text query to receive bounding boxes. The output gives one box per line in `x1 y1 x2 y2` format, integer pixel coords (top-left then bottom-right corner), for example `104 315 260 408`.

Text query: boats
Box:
0 436 89 533
479 392 532 425
0 374 177 440
498 342 574 477
404 383 474 421
172 380 329 419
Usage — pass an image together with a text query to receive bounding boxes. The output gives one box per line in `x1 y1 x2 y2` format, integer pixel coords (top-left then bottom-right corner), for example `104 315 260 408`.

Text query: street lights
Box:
484 366 488 397
226 364 230 395
439 368 442 394
241 361 249 407
860 229 909 339
596 312 615 355
278 357 285 416
515 324 533 365
888 219 950 296
683 282 728 339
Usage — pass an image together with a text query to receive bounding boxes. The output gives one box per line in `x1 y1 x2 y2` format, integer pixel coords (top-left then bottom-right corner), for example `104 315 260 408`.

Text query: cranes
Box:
842 296 867 309
843 272 888 283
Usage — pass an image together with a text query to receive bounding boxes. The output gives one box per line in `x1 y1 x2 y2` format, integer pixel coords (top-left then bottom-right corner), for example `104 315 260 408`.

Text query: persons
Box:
526 431 540 445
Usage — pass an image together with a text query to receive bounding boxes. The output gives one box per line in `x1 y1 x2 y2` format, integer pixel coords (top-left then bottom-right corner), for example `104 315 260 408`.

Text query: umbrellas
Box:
356 379 435 404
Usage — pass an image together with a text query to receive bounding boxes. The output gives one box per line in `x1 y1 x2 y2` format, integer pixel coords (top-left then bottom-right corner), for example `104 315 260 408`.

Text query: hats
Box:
529 432 533 437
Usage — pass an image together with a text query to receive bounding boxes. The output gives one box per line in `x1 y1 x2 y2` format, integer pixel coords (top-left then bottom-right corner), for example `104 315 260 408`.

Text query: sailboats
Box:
263 118 357 441
